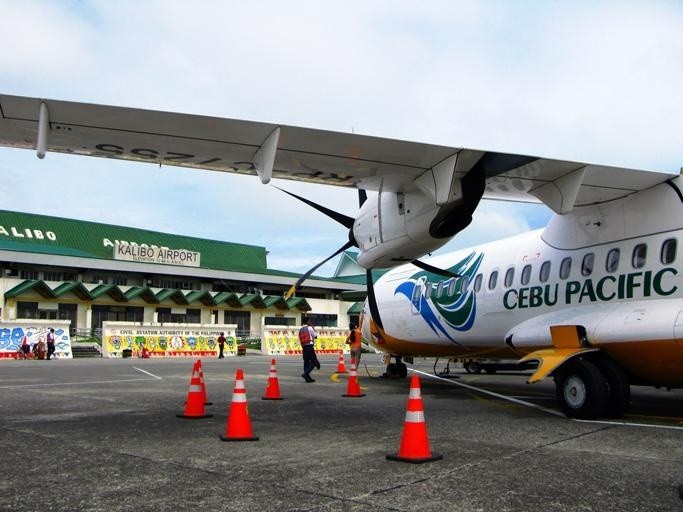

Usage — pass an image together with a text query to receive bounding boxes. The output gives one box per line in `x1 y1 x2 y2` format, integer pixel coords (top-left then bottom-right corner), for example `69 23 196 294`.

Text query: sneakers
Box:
301 372 315 382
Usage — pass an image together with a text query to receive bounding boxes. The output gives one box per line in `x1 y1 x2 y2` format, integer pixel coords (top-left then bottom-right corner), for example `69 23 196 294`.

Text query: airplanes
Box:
0 92 681 421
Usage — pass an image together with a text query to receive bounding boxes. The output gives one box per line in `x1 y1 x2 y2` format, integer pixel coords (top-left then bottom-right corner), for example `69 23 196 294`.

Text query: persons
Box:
299 317 321 383
218 332 228 359
345 309 364 368
45 329 54 360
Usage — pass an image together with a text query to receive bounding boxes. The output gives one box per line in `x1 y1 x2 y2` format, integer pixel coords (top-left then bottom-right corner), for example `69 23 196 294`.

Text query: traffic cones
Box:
341 357 365 398
334 350 348 373
176 359 213 419
218 369 259 442
261 358 284 400
384 374 443 464
